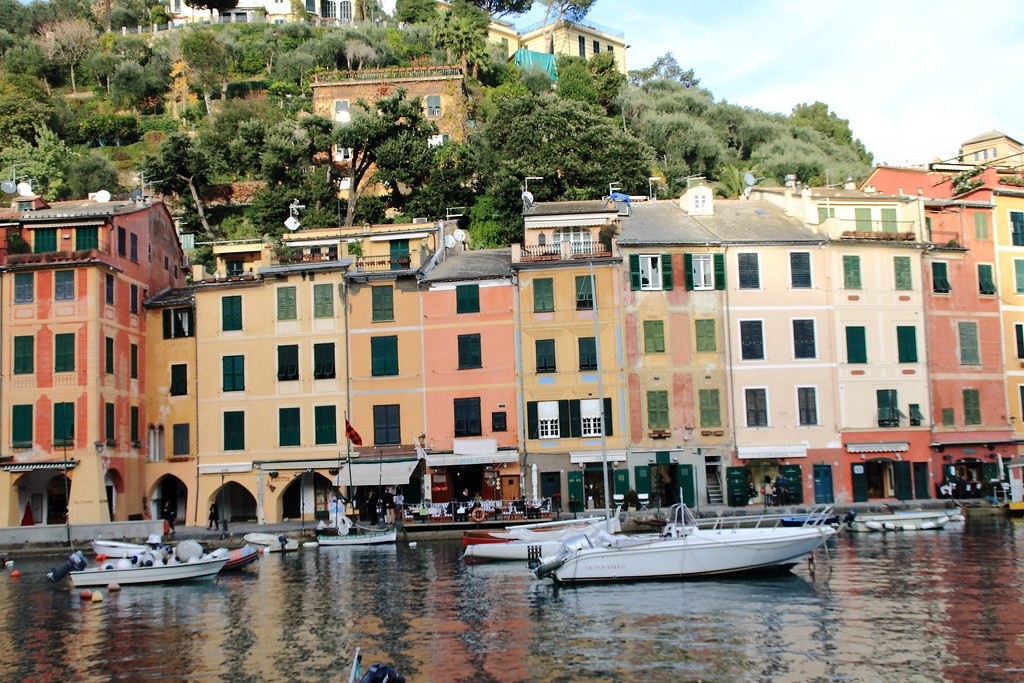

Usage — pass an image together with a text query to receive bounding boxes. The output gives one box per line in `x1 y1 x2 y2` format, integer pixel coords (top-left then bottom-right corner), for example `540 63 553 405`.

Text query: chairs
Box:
637 493 649 510
613 494 625 512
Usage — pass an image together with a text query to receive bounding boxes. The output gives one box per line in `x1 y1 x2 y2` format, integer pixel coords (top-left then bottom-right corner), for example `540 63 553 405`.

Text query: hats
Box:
521 496 526 499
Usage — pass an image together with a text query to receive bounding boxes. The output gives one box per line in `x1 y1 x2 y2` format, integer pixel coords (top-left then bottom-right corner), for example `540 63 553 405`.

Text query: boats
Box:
245 531 300 552
843 502 965 531
778 514 842 527
44 539 269 588
524 486 838 591
302 528 400 548
459 502 626 567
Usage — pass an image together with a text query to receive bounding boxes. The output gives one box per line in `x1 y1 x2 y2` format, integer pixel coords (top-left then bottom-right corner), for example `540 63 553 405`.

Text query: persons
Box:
447 498 458 521
465 499 482 515
512 496 549 519
419 503 427 522
163 501 175 534
207 498 219 530
940 475 977 497
382 488 396 525
764 476 773 506
365 490 378 526
773 474 785 506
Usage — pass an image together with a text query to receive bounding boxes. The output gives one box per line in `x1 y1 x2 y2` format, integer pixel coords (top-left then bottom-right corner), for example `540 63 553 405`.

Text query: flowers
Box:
952 179 985 196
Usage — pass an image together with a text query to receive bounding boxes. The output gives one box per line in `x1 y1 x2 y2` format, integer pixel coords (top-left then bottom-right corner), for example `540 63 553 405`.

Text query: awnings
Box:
425 449 519 466
735 445 807 459
332 457 423 486
932 439 1024 445
845 443 908 452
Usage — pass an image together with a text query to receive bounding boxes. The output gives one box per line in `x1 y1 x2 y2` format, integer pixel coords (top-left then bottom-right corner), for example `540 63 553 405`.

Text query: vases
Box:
205 275 254 283
398 259 409 264
649 433 671 438
702 431 725 436
522 255 561 262
972 183 981 189
365 260 398 266
571 252 611 259
843 231 906 240
954 189 964 195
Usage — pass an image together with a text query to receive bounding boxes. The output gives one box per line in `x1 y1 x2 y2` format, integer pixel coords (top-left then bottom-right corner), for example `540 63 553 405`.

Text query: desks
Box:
409 508 436 523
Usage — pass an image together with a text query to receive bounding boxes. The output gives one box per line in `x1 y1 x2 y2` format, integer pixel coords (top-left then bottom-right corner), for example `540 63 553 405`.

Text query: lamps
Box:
860 454 865 459
1010 417 1017 423
96 443 104 453
504 463 507 468
895 453 901 458
486 466 493 469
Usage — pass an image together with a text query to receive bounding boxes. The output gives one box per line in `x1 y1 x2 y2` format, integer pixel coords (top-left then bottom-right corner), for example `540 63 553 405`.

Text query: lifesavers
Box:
472 508 485 521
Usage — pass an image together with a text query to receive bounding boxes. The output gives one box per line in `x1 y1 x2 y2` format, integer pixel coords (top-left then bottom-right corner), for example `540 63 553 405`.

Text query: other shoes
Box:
524 517 526 519
528 517 531 519
768 503 771 506
214 528 219 530
206 528 211 530
532 517 534 518
170 531 175 535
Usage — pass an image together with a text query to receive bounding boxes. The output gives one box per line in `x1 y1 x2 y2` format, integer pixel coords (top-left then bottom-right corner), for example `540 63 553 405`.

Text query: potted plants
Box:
290 253 323 261
325 252 335 256
906 232 915 240
348 239 364 266
998 176 1024 187
274 248 295 260
949 240 959 247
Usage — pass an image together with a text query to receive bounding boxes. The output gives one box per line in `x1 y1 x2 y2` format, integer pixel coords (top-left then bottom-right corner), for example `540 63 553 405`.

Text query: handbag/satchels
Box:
760 487 765 494
170 511 177 520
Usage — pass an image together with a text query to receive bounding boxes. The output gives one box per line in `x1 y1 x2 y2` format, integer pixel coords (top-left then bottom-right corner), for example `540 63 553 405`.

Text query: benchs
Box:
539 502 551 518
442 509 452 523
403 510 414 524
432 508 442 523
513 506 524 520
502 506 512 520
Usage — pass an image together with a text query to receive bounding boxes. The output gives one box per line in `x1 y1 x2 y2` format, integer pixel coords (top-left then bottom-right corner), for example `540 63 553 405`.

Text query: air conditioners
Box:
183 255 190 266
